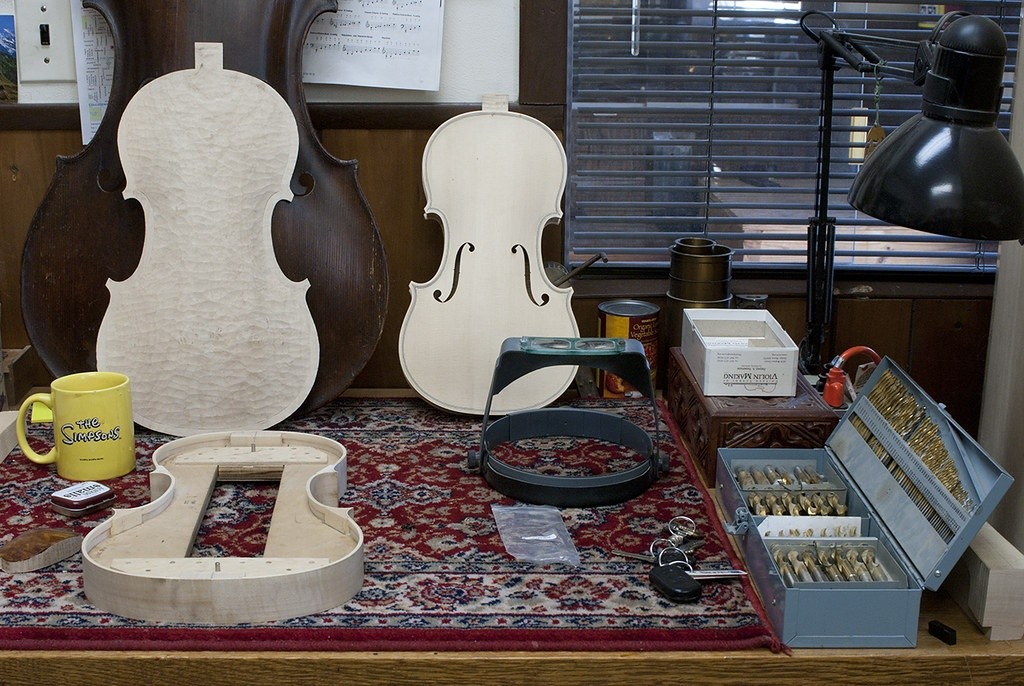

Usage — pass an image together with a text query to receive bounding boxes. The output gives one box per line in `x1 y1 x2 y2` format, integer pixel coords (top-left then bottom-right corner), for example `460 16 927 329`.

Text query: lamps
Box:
791 13 1024 391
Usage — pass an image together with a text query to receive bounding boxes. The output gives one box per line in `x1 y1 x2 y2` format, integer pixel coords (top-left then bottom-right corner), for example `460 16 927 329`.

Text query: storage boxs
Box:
667 355 1016 652
666 351 841 473
681 295 802 401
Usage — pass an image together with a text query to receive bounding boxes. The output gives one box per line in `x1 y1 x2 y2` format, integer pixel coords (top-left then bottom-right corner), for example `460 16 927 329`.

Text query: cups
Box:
16 372 136 481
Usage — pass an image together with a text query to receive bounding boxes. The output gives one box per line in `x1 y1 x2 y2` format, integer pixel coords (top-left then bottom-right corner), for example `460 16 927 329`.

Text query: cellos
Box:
20 1 391 438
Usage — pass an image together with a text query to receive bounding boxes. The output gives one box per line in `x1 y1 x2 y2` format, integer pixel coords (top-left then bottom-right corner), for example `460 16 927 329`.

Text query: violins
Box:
397 94 584 416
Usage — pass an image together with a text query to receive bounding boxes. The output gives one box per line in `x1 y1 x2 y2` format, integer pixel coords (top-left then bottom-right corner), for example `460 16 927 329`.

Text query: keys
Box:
609 514 750 602
611 547 660 565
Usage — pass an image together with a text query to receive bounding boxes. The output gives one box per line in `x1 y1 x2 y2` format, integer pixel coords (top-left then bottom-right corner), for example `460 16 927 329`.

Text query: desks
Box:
0 382 1024 686
